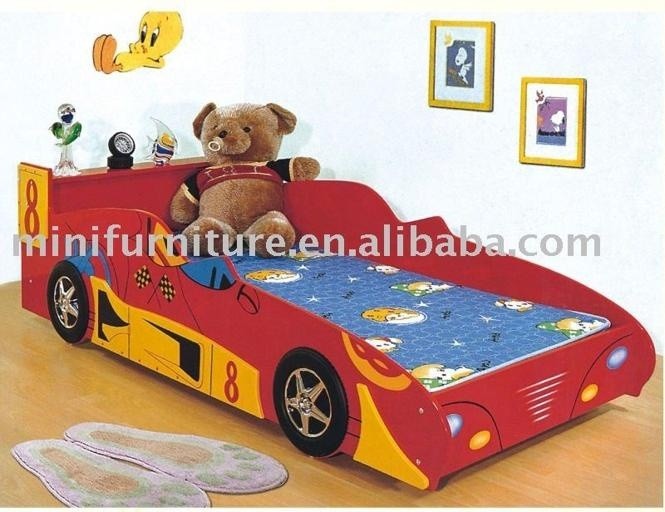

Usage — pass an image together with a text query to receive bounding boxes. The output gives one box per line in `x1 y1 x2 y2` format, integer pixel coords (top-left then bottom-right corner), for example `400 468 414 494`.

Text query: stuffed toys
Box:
168 100 322 260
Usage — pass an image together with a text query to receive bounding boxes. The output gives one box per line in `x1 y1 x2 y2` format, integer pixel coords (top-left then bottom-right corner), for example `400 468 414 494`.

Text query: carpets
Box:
12 420 292 507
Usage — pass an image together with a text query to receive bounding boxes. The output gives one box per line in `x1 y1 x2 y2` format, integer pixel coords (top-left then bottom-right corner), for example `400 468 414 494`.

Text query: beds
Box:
15 156 661 497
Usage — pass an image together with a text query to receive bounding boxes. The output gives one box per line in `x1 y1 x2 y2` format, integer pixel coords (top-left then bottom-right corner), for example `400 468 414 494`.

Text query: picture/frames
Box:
515 75 589 169
426 19 496 115
